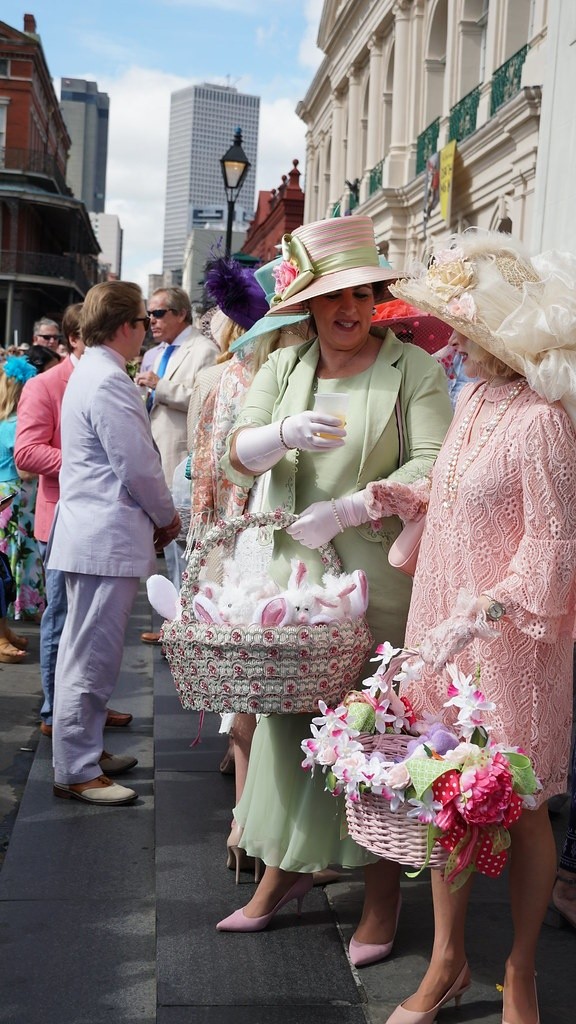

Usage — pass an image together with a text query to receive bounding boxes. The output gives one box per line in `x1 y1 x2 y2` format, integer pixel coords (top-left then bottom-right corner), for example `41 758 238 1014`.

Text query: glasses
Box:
121 316 150 332
36 333 61 341
395 328 415 346
147 308 182 319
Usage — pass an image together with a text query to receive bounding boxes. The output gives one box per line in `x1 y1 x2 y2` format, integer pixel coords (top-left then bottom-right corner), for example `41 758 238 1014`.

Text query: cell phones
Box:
0 492 18 505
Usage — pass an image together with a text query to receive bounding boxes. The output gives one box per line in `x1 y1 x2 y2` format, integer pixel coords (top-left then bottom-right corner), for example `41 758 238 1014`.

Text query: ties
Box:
144 345 175 415
126 364 134 384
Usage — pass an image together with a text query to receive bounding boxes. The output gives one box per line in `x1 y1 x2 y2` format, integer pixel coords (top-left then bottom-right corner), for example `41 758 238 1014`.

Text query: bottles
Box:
134 383 147 407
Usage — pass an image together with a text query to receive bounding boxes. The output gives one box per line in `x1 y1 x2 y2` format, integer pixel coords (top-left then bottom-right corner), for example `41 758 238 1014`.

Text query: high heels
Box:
6 628 29 650
208 734 576 1024
0 637 27 663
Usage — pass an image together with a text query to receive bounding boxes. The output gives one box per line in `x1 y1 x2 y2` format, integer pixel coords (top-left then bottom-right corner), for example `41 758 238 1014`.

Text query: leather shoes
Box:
40 705 143 809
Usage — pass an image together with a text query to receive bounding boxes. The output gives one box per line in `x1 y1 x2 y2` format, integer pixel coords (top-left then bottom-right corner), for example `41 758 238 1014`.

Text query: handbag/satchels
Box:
387 509 426 576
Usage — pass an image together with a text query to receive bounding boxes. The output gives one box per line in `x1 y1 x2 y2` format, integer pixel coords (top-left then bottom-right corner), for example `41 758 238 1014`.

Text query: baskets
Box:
145 506 374 716
298 638 542 896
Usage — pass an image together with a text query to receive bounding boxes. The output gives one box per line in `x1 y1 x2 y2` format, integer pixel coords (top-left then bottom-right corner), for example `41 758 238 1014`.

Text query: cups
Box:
314 393 347 439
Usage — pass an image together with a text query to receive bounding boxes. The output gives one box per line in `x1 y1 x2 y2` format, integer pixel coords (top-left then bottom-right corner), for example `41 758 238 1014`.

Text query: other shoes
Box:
140 632 162 646
22 608 43 625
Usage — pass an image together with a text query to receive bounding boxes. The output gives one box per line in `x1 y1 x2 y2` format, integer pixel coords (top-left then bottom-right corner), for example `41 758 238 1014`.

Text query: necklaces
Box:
441 375 526 509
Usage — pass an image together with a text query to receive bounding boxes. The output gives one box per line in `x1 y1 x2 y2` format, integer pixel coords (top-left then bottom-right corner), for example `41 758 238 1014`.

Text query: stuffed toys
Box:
191 595 286 629
146 574 222 628
221 557 268 628
322 570 368 617
284 558 339 624
364 711 459 770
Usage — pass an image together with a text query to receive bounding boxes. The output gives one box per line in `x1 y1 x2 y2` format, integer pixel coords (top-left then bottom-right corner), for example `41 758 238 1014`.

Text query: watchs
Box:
487 595 504 622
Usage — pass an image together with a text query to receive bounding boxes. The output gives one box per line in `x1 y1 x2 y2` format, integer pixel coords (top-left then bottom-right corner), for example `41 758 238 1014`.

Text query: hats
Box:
387 225 576 429
228 256 311 353
203 237 271 329
18 343 30 351
266 215 422 317
370 302 452 356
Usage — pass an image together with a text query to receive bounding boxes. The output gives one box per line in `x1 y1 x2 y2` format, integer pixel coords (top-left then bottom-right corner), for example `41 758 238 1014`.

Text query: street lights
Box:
219 127 252 257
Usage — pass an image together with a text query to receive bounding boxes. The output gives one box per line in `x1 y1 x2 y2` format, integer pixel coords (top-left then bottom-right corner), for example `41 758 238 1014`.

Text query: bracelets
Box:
332 497 345 534
280 416 295 450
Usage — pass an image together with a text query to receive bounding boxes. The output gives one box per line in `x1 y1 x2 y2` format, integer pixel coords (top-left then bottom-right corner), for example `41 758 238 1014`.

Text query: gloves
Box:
420 584 504 675
285 488 374 550
236 410 347 473
362 477 431 522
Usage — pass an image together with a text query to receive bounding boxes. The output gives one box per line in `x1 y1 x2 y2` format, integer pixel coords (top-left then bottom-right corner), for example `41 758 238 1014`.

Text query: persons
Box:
0 254 464 883
42 283 183 805
365 236 576 1024
212 211 453 968
